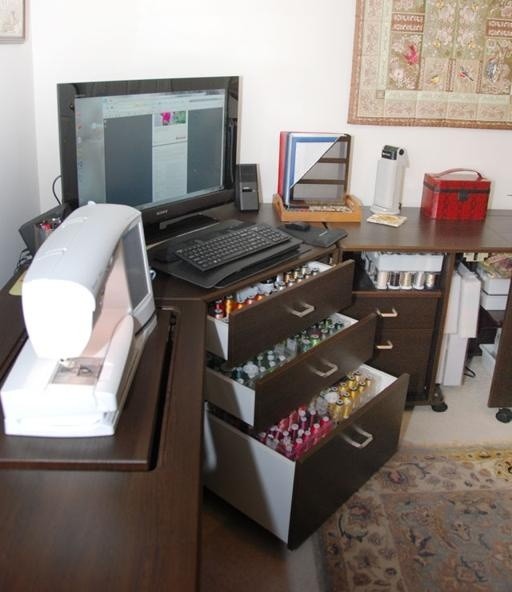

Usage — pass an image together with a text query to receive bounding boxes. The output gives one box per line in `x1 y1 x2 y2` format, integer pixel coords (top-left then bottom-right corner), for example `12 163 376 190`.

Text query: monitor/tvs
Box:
54 75 242 248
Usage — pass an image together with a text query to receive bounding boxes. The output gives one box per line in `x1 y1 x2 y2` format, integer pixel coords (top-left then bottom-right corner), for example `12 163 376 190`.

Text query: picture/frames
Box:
347 0 512 129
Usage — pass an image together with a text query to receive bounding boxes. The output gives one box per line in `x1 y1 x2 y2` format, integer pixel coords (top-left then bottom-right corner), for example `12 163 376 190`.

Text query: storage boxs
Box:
420 167 490 221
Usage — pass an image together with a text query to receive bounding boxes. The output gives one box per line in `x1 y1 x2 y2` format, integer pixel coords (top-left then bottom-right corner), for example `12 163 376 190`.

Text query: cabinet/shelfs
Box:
332 251 458 404
201 260 410 550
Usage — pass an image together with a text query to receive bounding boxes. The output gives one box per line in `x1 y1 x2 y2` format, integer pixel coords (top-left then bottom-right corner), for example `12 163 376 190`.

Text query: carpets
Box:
318 444 512 592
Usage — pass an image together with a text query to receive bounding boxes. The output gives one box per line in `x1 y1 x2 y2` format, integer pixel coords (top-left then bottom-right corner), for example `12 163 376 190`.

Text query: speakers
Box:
17 203 73 251
235 162 261 215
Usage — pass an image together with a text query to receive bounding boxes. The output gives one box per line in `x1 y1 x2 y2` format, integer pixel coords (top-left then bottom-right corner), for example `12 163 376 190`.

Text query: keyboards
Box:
173 223 293 272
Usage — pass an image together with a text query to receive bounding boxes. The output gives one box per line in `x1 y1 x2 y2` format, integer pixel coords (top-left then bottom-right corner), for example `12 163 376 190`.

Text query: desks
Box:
325 205 512 254
151 196 337 299
0 258 208 592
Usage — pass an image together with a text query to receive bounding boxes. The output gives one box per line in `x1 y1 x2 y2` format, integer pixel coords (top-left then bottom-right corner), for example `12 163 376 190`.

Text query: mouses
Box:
286 221 313 232
